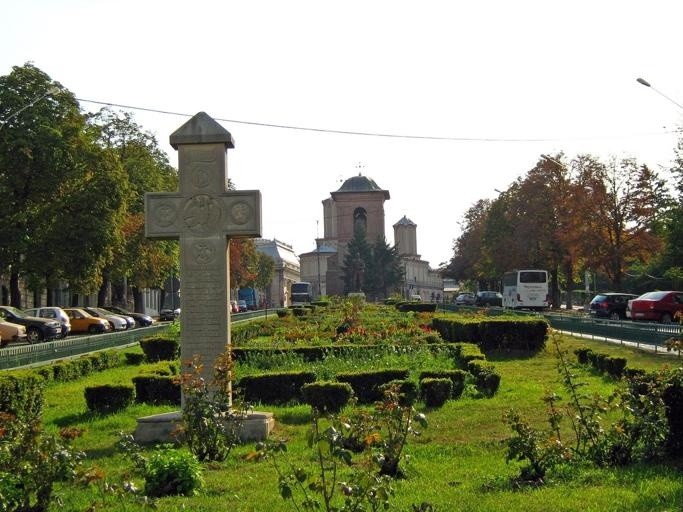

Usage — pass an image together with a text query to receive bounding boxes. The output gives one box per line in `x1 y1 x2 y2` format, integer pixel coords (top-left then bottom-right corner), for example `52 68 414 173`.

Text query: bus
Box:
499 268 551 311
290 282 312 309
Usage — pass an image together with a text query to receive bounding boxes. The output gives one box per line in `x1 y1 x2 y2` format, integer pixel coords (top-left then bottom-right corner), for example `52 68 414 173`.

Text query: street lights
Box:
633 74 683 110
314 219 322 295
0 85 64 136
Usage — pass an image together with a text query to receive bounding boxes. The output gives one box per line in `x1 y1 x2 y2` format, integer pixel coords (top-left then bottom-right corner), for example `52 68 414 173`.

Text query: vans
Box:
159 301 177 320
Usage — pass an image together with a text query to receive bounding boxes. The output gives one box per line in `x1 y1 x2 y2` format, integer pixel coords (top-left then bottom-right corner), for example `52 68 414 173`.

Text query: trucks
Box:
235 287 259 312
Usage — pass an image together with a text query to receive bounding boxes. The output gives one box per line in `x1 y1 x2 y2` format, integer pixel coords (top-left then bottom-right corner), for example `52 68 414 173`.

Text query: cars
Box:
410 294 422 301
453 290 504 307
587 290 640 323
173 308 181 319
230 298 248 314
0 304 156 347
625 289 683 324
